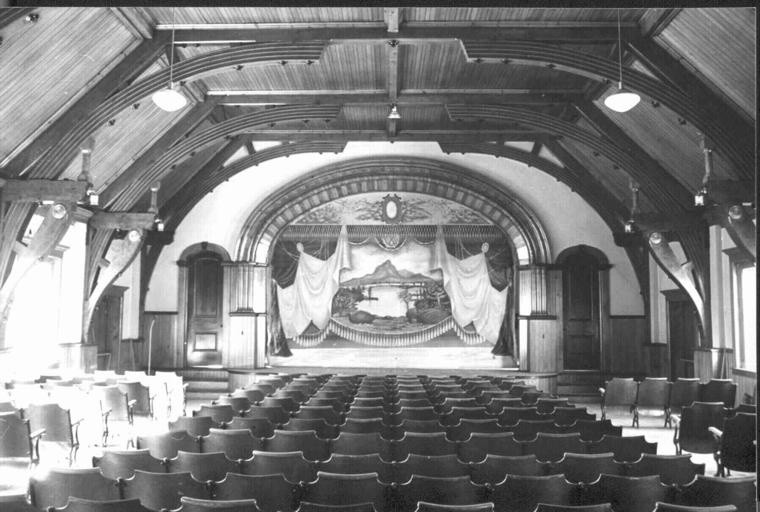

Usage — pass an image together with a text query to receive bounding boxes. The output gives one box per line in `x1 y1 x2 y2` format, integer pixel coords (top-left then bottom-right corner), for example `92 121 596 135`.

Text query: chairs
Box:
1 369 758 512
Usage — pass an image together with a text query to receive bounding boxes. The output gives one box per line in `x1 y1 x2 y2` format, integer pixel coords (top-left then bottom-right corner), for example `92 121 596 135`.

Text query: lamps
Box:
149 7 190 114
386 104 402 120
602 9 644 113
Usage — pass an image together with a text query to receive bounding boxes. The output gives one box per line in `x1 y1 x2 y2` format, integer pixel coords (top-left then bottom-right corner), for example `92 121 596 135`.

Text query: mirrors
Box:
380 191 402 222
